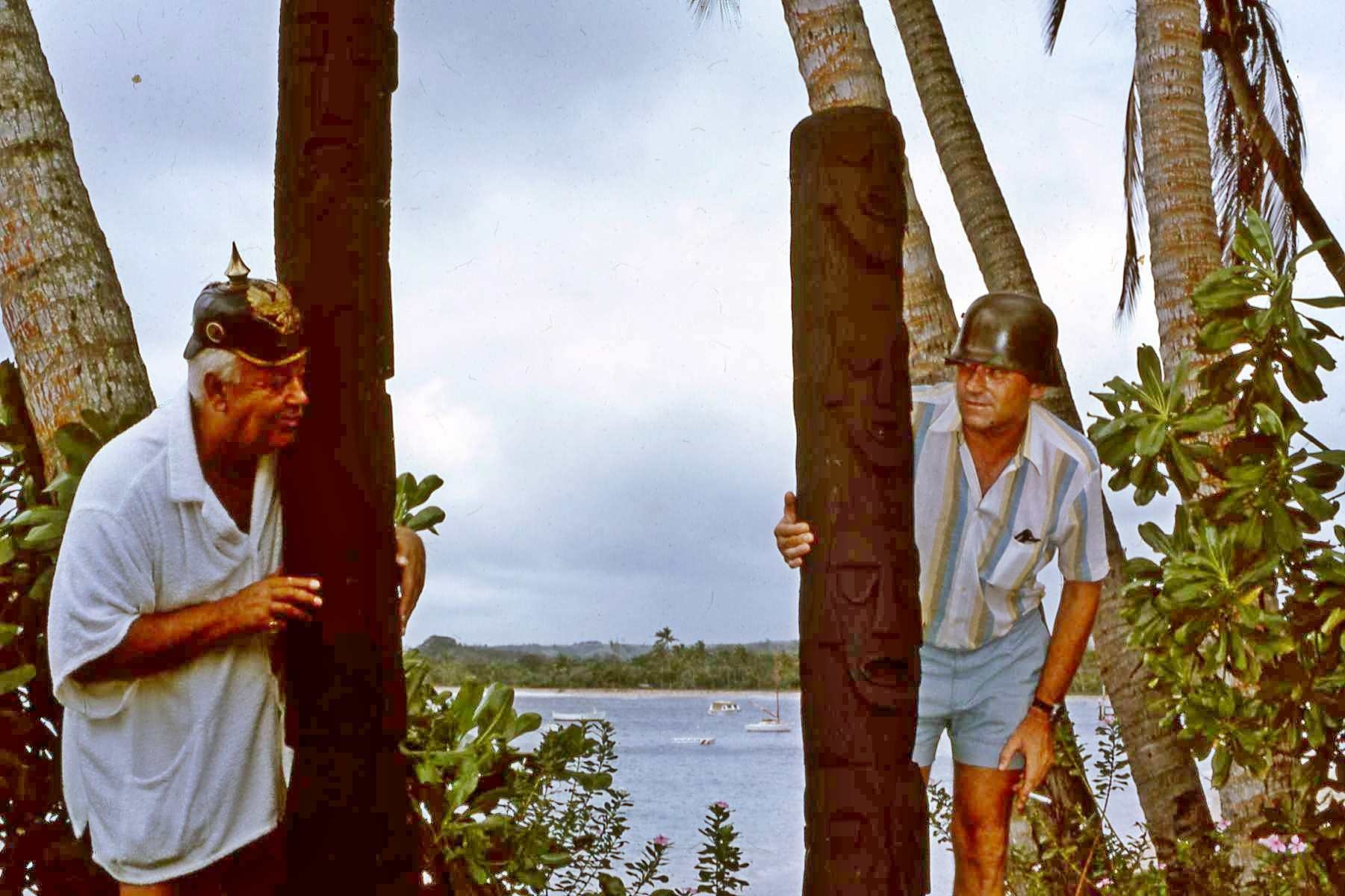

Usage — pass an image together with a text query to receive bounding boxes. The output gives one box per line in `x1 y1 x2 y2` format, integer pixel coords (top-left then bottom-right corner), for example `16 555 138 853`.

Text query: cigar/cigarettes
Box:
1028 792 1051 804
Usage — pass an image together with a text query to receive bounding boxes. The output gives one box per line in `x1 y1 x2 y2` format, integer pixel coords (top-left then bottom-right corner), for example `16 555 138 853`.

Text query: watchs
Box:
1032 697 1065 720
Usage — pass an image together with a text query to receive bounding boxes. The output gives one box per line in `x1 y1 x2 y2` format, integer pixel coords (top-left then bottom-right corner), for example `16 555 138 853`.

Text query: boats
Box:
1100 705 1116 725
707 701 736 715
552 711 605 721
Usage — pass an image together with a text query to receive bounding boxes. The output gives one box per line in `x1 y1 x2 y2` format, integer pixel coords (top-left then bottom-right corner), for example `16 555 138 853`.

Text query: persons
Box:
45 240 427 896
774 293 1111 896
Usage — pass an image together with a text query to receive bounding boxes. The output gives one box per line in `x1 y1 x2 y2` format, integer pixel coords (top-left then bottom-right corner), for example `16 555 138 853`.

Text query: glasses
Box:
1014 529 1041 543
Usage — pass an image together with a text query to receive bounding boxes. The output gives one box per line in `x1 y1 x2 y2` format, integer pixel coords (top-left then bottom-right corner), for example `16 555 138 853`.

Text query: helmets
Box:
945 293 1058 383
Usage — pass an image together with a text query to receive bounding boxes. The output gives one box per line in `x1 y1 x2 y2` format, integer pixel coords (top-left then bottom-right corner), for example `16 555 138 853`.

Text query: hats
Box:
183 241 311 367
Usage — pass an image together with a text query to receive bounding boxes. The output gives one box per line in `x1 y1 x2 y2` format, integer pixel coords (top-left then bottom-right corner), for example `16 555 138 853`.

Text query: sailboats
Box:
745 645 791 732
